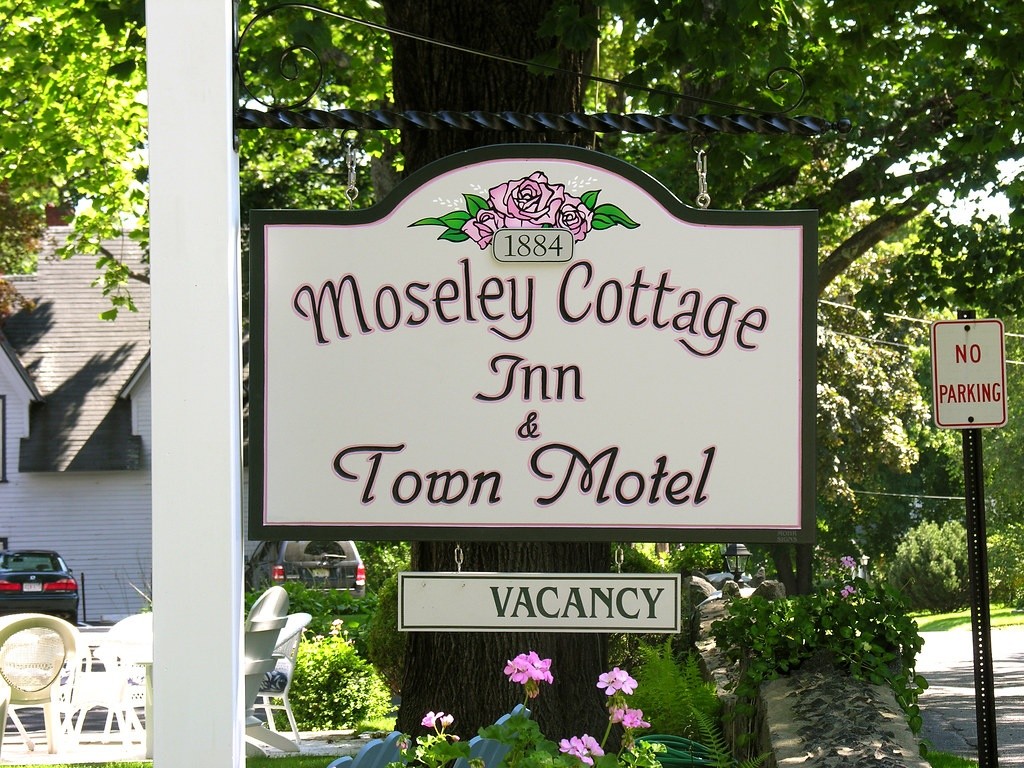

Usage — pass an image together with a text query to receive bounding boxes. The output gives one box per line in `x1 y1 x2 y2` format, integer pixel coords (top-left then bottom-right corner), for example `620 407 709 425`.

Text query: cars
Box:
245 540 366 600
0 548 79 625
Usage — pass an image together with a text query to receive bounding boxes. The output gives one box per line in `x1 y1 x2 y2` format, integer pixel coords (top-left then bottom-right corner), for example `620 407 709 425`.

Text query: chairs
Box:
0 617 77 754
35 564 50 569
0 612 92 746
244 585 301 758
254 611 312 744
94 613 152 745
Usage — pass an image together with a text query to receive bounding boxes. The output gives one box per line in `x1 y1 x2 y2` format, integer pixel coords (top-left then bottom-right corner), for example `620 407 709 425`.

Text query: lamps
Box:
721 542 753 589
